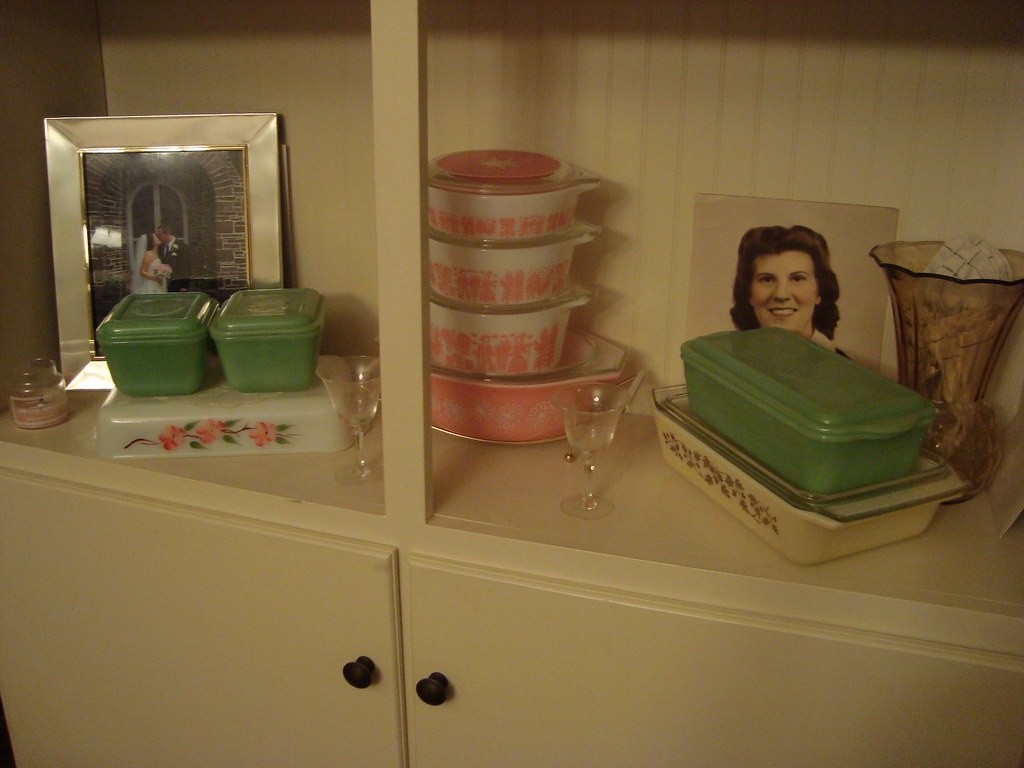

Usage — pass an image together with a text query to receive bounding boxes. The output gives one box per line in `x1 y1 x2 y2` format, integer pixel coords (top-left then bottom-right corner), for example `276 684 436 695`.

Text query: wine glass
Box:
315 355 381 485
552 381 631 521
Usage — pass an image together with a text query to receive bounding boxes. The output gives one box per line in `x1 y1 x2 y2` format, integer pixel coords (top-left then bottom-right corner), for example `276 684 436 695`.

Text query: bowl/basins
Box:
427 144 644 444
680 326 936 495
207 289 329 392
96 287 220 395
651 382 971 569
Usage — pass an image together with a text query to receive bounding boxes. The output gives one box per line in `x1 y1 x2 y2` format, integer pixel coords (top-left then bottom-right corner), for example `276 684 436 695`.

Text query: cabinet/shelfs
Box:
1 468 1024 768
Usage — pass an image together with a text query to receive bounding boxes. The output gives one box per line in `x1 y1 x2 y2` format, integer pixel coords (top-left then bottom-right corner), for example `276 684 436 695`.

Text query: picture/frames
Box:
44 112 285 396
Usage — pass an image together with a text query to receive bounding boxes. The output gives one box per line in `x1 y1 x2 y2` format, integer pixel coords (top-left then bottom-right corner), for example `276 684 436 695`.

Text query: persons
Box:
726 221 854 367
126 225 200 294
